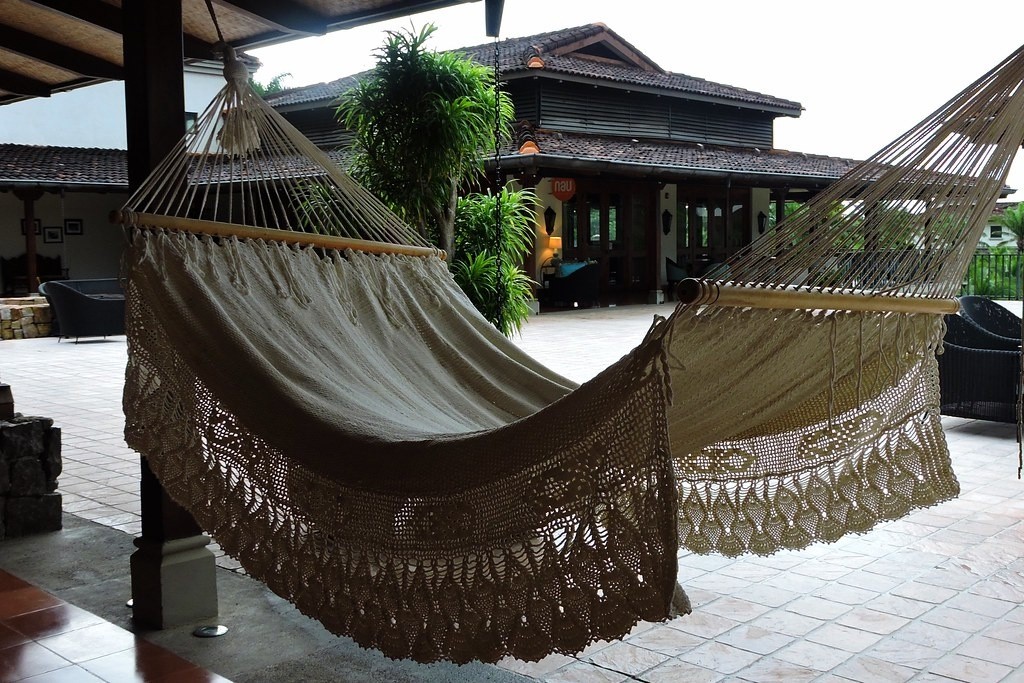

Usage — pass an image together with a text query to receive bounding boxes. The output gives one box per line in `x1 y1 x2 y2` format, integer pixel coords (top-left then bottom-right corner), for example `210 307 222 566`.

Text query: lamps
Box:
542 205 557 236
757 211 766 233
660 209 674 235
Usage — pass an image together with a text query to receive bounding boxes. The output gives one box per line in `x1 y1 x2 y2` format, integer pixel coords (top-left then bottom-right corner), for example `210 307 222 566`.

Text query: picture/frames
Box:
20 218 41 235
43 225 63 243
64 218 84 235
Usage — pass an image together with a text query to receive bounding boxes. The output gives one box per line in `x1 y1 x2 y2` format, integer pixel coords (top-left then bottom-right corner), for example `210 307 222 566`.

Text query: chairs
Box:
936 295 1023 442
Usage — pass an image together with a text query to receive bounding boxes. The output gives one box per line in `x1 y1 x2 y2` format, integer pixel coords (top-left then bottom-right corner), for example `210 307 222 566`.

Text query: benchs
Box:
1 252 126 343
